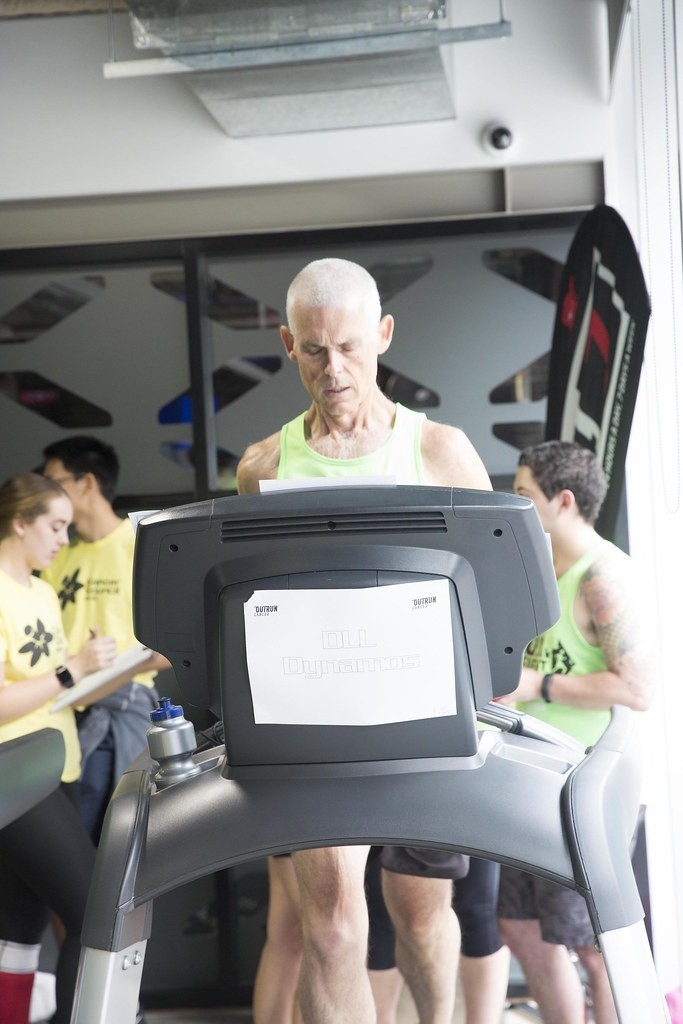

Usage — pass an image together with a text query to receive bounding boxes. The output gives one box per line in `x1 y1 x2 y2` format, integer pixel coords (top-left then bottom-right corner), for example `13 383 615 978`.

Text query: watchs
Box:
56 666 75 689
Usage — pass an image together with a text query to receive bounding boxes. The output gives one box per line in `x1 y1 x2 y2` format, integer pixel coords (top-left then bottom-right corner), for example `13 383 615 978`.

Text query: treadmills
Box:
70 480 669 1023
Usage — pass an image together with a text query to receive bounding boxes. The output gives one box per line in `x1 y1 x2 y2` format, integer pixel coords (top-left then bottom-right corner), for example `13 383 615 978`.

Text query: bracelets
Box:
540 673 555 704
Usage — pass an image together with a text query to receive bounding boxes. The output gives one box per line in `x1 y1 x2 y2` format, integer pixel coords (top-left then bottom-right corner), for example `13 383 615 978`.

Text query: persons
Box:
236 254 498 1024
0 473 117 1024
254 851 313 1024
34 436 174 1024
361 839 509 1024
502 442 659 1024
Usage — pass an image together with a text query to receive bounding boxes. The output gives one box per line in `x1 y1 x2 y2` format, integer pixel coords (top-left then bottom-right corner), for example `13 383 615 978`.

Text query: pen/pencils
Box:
88 628 98 640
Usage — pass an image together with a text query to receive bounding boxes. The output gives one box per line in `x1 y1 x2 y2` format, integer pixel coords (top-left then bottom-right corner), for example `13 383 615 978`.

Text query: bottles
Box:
146 698 202 794
